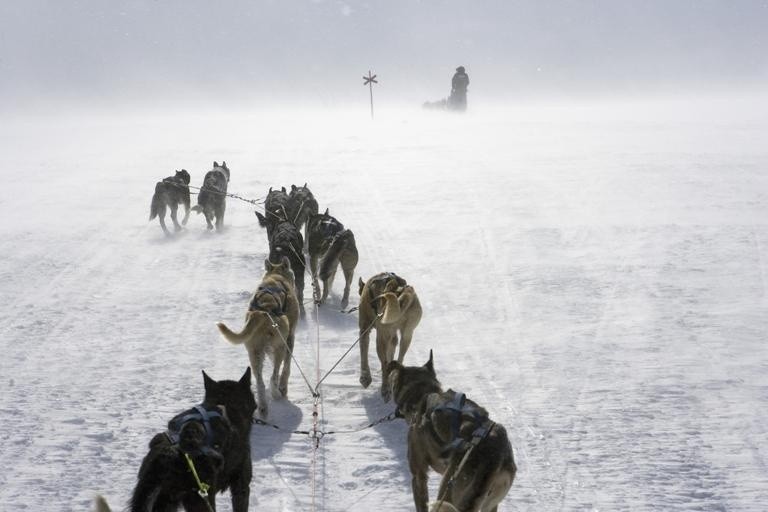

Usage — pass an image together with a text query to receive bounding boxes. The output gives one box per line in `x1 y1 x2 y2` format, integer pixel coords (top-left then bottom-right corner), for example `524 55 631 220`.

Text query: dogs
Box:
254 185 359 321
216 255 300 419
190 161 231 234
93 365 259 512
148 169 191 239
384 350 518 512
357 272 423 403
